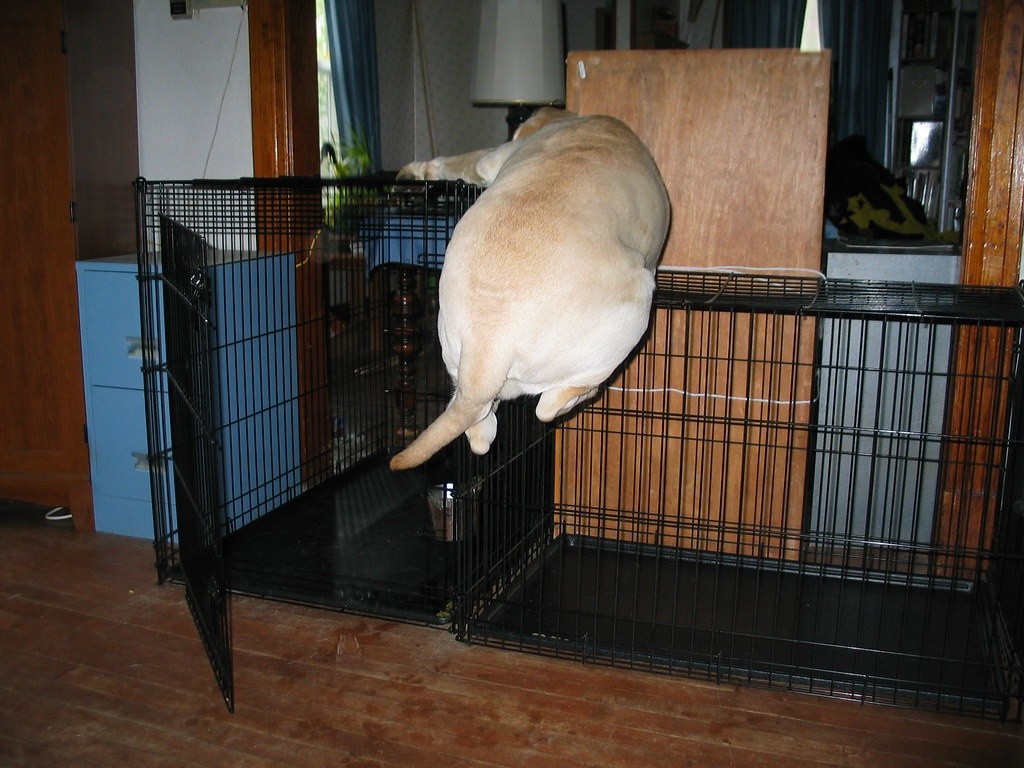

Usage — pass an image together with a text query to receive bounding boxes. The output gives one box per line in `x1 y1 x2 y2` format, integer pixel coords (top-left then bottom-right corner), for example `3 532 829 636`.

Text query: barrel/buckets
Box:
428 483 478 542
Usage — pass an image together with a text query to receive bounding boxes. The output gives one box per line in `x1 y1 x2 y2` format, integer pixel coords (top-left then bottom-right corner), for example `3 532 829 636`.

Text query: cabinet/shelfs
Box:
0 0 141 537
76 250 299 548
890 0 977 234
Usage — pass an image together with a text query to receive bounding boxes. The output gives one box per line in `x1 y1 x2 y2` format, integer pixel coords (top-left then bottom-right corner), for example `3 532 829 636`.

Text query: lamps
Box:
472 0 566 143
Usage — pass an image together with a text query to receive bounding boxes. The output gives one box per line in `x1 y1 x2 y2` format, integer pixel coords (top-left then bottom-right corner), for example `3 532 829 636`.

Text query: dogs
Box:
389 109 671 468
